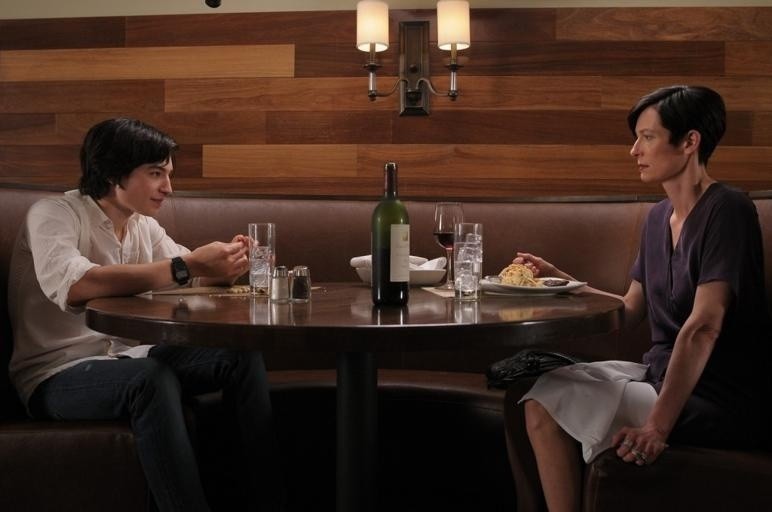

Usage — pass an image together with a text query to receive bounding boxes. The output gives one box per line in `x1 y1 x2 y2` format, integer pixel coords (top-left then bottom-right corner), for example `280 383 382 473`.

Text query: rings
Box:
631 448 641 459
641 453 648 462
623 439 633 448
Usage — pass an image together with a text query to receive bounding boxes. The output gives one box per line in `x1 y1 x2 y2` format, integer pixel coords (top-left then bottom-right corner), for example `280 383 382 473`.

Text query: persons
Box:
512 84 767 512
7 118 261 512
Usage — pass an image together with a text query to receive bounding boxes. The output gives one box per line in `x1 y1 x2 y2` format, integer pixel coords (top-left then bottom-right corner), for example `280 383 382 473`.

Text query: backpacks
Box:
486 348 576 390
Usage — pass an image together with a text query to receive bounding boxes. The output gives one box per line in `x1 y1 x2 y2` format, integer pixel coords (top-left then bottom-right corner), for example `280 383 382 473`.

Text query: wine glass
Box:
432 203 466 292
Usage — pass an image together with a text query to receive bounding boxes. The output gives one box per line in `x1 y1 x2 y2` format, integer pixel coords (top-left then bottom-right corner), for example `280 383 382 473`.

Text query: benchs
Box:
1 188 771 508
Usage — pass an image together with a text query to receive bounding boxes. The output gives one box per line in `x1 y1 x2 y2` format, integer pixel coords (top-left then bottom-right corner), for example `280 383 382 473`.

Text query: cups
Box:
248 222 276 297
452 223 486 302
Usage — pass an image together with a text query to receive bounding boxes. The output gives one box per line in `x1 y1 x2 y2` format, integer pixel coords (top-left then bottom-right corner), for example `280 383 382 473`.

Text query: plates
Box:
353 266 446 286
479 276 589 296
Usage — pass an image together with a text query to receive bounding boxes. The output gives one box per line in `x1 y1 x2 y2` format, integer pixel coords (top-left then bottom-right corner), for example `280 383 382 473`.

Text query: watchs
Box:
172 257 189 285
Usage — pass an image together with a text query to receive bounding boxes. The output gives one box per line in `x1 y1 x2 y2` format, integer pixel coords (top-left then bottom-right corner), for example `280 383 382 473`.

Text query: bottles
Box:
271 263 313 304
370 162 409 307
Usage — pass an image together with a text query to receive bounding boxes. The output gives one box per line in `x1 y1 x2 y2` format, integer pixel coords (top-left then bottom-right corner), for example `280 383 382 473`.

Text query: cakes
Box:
498 263 537 287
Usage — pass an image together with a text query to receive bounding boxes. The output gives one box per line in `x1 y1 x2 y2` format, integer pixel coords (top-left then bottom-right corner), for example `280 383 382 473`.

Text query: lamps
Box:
355 2 470 116
